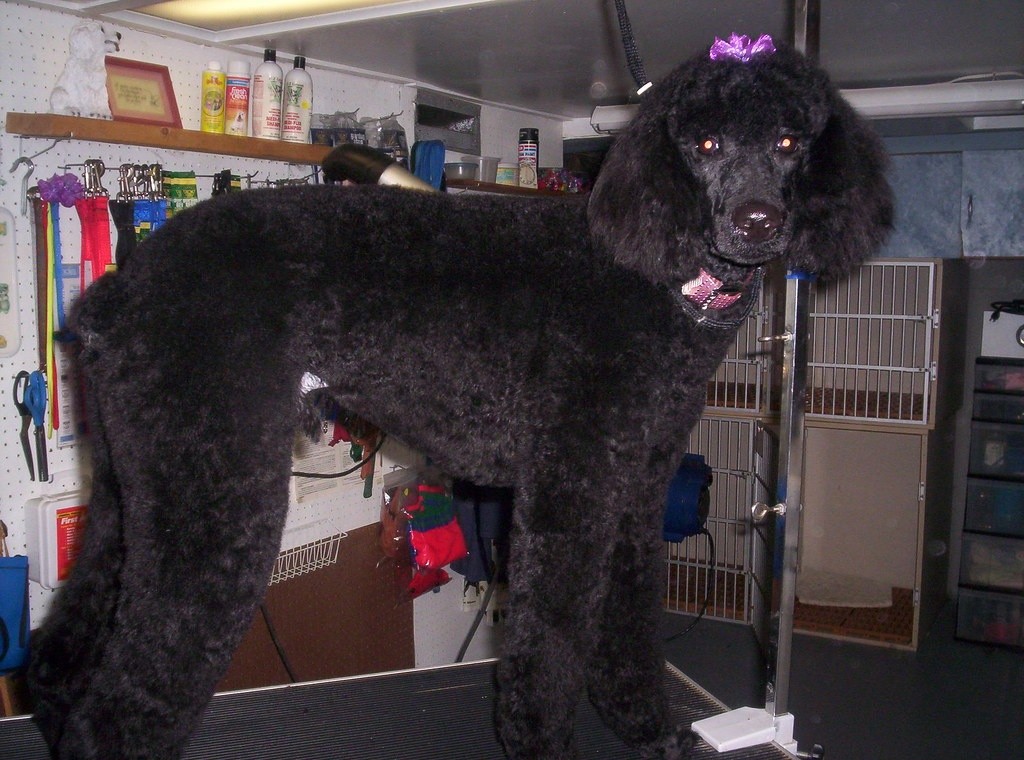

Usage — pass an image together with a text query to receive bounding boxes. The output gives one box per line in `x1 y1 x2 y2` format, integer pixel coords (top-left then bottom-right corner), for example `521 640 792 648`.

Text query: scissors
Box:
13 371 36 482
24 370 48 482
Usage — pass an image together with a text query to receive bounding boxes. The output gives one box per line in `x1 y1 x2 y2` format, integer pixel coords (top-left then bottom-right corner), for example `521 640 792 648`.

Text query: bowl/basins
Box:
461 157 500 183
443 163 478 179
495 163 520 186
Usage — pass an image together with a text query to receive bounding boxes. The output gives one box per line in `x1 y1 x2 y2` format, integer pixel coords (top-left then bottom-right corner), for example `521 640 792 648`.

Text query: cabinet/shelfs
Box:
933 256 1024 649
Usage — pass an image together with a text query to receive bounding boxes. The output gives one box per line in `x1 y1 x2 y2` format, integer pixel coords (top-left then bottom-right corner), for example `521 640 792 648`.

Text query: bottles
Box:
201 62 226 134
518 128 539 189
227 61 251 135
252 49 283 141
283 57 313 144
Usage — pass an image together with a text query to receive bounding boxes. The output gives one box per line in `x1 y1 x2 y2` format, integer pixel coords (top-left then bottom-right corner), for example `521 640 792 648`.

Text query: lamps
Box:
591 78 1024 134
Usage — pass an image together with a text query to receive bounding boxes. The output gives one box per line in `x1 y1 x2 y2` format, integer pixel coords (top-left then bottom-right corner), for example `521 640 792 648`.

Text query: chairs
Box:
658 452 717 644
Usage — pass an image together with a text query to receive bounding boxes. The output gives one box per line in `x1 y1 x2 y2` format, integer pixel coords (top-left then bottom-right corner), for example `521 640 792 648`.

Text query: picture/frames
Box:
105 55 182 129
